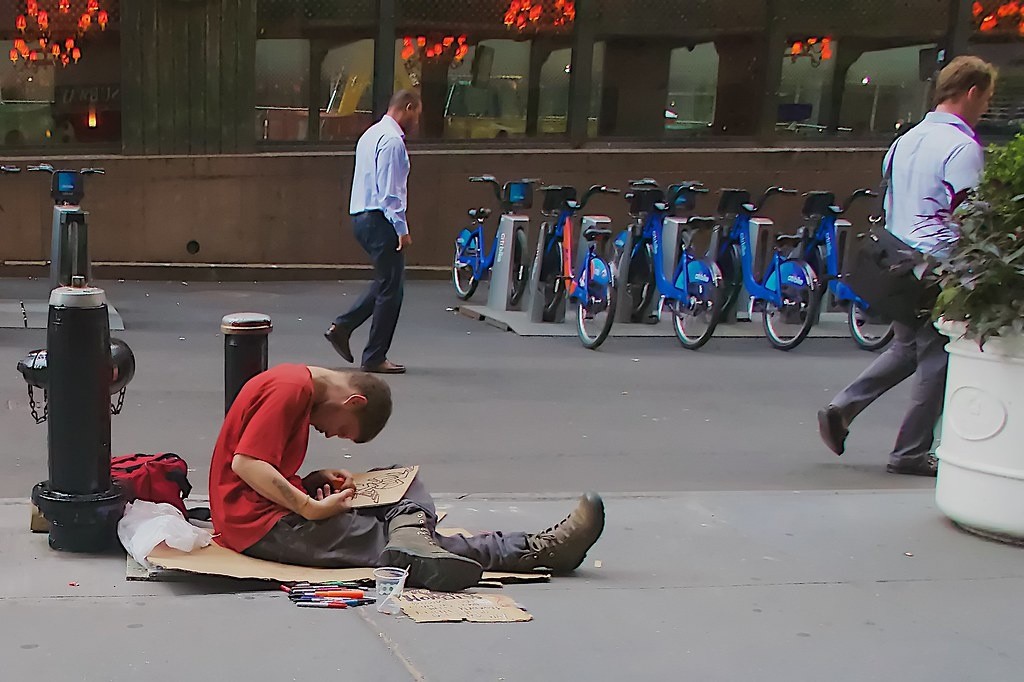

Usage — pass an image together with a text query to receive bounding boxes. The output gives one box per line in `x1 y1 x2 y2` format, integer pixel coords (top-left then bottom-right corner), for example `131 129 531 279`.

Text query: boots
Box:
380 512 484 593
519 492 604 577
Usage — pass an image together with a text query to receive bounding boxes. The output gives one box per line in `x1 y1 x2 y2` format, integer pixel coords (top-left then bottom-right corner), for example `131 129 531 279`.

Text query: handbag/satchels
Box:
839 222 950 330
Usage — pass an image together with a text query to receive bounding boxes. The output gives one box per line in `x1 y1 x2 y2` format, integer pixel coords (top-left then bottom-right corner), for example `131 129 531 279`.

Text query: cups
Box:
374 567 408 615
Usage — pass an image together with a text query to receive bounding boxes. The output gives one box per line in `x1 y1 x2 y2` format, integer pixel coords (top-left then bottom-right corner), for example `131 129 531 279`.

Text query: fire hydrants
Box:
17 278 137 552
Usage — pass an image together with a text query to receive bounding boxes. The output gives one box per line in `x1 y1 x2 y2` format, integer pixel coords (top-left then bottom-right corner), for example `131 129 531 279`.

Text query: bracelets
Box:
296 494 310 515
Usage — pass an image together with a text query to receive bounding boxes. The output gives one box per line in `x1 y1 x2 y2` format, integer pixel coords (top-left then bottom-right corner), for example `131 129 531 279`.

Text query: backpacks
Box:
108 452 212 520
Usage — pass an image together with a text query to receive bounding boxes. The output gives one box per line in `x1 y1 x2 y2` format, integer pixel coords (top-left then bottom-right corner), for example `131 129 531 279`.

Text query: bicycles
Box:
706 187 819 351
609 178 725 350
537 185 622 349
775 190 895 352
26 163 106 277
452 174 545 304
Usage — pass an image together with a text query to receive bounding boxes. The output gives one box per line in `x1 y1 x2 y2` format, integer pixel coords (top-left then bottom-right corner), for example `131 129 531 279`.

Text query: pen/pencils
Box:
280 577 377 610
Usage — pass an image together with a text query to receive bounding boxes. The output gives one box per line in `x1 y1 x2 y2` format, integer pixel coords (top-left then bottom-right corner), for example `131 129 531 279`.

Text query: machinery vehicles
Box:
319 38 599 141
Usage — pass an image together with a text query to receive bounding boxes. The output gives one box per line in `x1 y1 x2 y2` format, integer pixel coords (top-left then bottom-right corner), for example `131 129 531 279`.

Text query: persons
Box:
323 88 423 373
817 56 996 477
208 364 605 593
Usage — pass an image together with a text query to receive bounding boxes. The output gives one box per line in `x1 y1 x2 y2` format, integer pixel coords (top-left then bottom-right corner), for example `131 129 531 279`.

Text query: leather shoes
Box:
323 322 355 364
819 403 849 455
887 453 940 477
360 357 407 374
